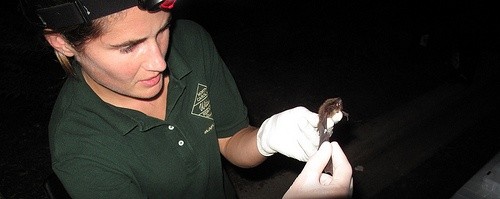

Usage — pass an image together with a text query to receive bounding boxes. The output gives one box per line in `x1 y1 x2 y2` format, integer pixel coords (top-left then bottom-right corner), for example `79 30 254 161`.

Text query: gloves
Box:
256 106 343 162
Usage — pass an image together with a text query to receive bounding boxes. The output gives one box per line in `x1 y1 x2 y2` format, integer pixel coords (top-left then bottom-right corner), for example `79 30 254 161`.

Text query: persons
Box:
30 0 354 199
